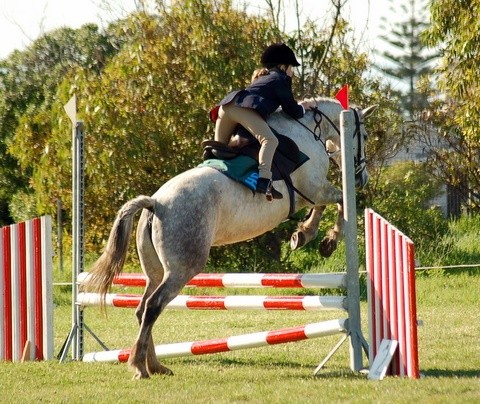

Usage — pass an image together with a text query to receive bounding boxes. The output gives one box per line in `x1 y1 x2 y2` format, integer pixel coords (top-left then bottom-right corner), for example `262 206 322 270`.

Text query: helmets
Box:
260 43 300 67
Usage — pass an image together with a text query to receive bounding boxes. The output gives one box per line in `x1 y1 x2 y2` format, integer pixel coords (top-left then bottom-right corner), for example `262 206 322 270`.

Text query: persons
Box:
209 44 318 199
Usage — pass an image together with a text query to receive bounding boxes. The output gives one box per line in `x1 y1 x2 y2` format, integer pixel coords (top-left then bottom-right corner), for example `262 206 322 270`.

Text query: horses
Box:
78 97 380 381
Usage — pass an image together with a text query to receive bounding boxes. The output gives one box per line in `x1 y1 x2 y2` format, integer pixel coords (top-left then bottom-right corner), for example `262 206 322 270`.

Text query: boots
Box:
256 177 283 199
